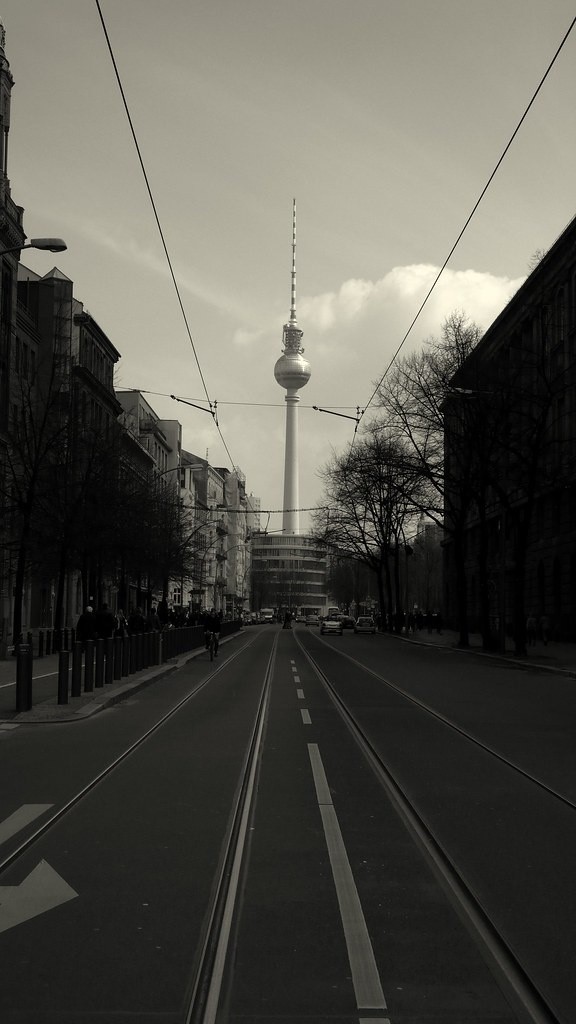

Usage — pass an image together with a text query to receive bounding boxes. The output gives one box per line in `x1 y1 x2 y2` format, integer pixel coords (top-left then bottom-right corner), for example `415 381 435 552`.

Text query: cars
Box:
296 615 306 624
354 616 376 635
329 612 356 629
305 615 319 627
241 608 275 626
321 616 343 636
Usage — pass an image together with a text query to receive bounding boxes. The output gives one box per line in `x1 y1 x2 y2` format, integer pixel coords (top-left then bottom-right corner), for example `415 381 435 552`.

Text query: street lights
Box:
179 517 225 605
126 463 204 620
200 531 244 600
242 559 268 610
215 544 249 588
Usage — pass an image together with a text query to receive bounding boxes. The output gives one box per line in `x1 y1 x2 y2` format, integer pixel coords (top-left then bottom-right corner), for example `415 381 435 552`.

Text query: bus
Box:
327 606 340 616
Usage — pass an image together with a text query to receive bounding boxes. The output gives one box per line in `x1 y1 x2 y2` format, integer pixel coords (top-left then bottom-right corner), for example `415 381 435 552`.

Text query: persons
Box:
539 613 550 646
75 603 244 654
527 614 536 646
203 611 219 657
272 612 283 624
377 609 442 634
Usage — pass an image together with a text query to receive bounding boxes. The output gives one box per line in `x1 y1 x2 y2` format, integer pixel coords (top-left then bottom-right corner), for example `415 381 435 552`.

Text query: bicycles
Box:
203 631 220 662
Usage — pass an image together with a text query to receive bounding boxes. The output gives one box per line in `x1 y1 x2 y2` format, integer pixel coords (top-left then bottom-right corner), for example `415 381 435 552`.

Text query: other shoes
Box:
215 649 217 653
206 644 210 649
544 640 547 646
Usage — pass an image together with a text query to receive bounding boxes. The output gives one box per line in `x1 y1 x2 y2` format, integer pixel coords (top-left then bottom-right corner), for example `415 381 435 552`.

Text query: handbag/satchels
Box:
528 620 536 630
542 621 550 631
116 617 120 630
